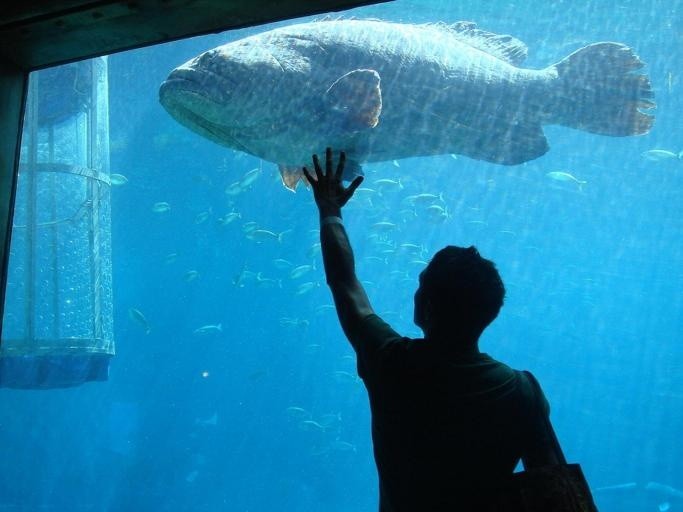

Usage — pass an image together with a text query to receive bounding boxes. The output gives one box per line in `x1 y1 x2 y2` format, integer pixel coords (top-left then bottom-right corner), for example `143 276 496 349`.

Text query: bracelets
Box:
316 214 347 231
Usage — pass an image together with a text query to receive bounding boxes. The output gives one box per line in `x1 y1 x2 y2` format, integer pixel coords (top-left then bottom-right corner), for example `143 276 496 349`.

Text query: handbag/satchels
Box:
509 369 599 512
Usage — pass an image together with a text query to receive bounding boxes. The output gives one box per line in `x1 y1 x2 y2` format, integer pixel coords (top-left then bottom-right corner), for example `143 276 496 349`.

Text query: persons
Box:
301 147 583 512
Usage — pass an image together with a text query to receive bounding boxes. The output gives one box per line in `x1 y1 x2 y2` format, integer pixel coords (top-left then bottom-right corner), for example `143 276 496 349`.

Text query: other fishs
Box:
151 168 486 337
